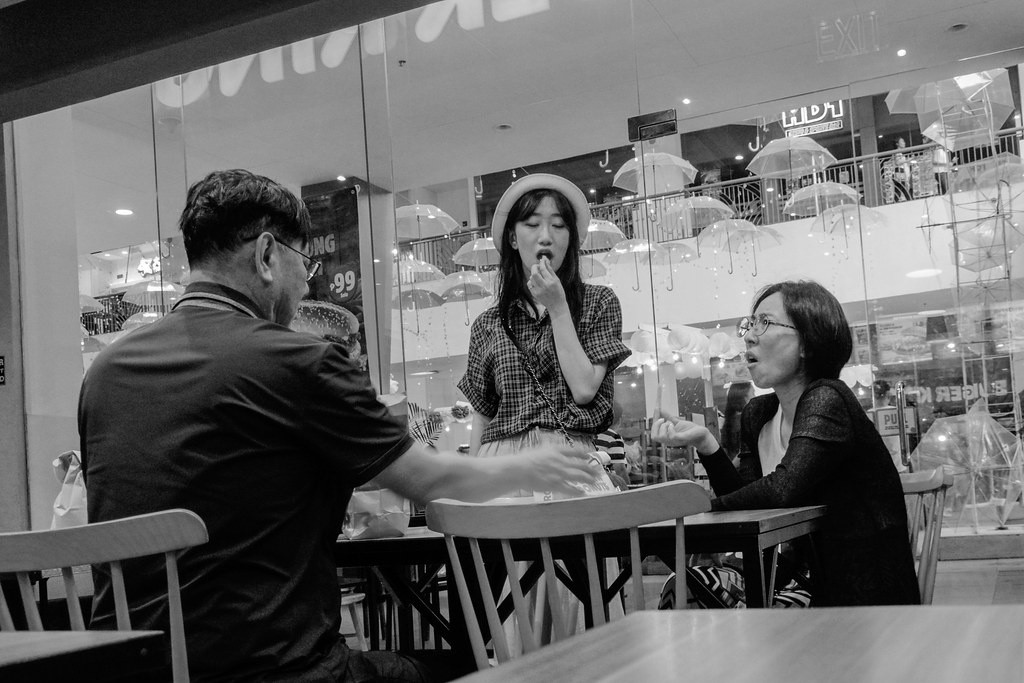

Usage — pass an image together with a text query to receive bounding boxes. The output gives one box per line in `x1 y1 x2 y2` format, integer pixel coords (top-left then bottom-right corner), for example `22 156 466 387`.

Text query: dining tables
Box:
335 505 828 683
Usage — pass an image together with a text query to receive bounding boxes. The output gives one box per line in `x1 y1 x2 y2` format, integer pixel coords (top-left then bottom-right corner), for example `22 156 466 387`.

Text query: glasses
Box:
736 314 795 338
273 236 321 281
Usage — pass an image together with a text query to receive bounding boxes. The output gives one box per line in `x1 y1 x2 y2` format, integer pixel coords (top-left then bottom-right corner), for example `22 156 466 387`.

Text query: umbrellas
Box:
74 68 1024 543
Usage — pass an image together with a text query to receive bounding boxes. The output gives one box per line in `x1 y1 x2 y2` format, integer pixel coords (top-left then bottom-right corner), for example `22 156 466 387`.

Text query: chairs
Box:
0 509 210 683
901 465 954 605
426 478 712 672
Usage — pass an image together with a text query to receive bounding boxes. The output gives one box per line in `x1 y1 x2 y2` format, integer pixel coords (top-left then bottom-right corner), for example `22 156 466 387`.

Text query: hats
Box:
491 173 591 254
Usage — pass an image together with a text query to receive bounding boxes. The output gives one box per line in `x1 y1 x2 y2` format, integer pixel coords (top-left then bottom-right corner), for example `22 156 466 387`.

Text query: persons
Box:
893 138 912 195
717 379 759 474
925 129 953 197
455 173 631 655
646 280 922 612
864 380 909 476
74 168 599 683
592 397 632 493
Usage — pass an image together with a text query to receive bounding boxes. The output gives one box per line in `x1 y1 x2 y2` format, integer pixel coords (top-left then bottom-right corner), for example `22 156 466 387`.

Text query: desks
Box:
0 630 165 683
446 605 1024 683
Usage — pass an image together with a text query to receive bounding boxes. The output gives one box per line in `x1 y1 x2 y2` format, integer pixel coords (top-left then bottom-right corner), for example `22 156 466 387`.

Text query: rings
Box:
527 285 533 290
